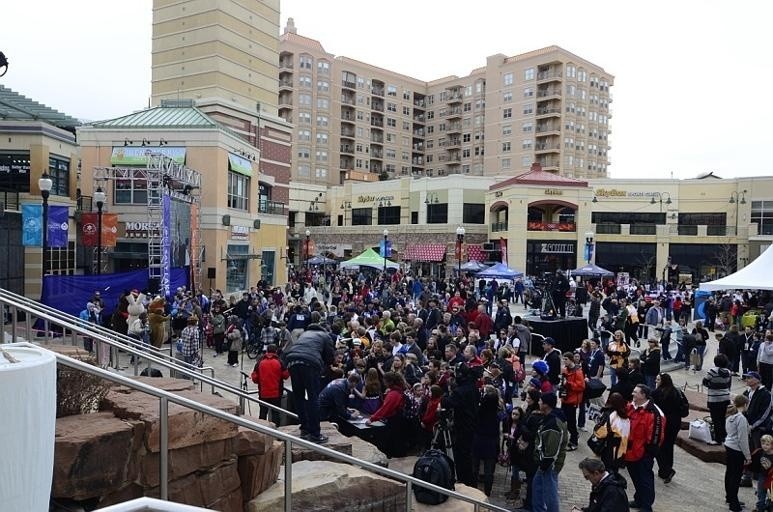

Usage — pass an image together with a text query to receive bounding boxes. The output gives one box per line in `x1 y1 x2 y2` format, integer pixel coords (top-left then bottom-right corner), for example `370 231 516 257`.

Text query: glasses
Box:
542 343 547 345
415 389 423 392
584 473 593 479
453 311 457 312
393 359 400 362
499 333 505 335
480 355 485 358
512 412 519 416
427 342 434 345
615 335 620 336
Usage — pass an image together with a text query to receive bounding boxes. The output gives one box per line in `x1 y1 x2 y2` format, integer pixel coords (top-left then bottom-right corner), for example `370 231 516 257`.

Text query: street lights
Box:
305 230 310 269
456 225 465 280
585 231 594 264
382 228 389 271
36 168 53 278
91 184 106 274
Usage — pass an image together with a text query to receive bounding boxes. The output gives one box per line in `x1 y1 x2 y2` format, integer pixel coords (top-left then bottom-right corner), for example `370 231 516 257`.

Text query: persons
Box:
420 385 443 450
626 358 647 383
751 435 773 512
504 408 532 496
331 320 524 380
523 390 545 430
626 384 666 511
531 392 567 510
703 354 733 445
286 323 330 444
650 372 689 483
478 382 502 496
593 393 631 478
290 257 525 319
251 345 289 427
438 362 480 488
366 373 405 458
79 275 289 361
317 374 360 433
526 359 556 392
568 456 630 511
556 352 586 451
405 383 425 453
526 265 773 358
725 396 752 512
741 370 772 447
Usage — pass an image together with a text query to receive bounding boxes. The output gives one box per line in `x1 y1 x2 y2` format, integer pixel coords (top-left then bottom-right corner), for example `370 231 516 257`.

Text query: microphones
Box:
239 370 251 378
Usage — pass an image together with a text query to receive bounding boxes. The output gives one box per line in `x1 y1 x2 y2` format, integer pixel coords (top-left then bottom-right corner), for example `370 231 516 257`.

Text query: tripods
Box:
541 286 558 317
241 379 251 415
429 422 457 482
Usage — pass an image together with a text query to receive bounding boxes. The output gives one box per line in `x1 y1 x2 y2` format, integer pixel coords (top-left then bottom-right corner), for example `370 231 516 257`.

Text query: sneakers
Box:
668 358 673 362
551 316 557 319
696 367 702 371
674 360 677 363
566 444 578 451
233 363 239 367
663 358 667 361
558 315 565 319
752 508 766 512
634 339 640 348
664 469 675 484
214 353 218 357
629 501 644 508
766 503 772 512
301 432 310 441
658 470 668 479
685 365 690 370
225 362 230 366
728 507 748 512
578 426 588 432
310 434 328 444
726 499 745 507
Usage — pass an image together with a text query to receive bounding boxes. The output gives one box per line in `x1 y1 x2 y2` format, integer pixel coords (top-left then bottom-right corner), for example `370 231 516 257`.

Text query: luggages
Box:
587 396 607 410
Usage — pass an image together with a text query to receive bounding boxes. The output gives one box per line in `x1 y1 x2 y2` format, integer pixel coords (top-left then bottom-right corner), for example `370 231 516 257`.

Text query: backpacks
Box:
413 451 456 505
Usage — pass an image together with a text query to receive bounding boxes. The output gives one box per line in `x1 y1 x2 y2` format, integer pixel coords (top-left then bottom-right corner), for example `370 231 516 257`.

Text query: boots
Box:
504 479 513 495
507 480 521 500
739 479 752 487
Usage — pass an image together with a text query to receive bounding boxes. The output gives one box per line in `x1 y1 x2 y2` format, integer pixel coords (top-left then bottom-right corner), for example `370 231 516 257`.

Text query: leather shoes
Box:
707 439 721 445
721 438 725 442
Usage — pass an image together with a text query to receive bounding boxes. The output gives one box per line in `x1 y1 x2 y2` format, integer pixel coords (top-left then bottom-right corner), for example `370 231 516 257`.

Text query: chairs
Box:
240 371 259 417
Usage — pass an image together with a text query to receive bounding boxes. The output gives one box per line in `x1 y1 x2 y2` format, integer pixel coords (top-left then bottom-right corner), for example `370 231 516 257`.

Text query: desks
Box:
283 374 387 450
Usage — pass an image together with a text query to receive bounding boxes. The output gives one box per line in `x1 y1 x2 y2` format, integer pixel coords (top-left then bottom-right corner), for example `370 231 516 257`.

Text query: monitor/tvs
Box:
169 200 192 267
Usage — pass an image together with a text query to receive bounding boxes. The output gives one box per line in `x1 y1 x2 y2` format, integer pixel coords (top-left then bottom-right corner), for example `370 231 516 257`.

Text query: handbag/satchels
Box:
517 410 543 452
498 434 512 468
143 327 149 344
587 414 613 458
688 418 711 444
676 387 689 418
703 416 715 439
690 348 701 366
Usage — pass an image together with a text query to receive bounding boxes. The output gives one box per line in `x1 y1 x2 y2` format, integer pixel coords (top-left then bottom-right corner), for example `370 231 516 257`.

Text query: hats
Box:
352 338 362 346
591 338 600 347
228 314 240 323
744 371 761 380
87 302 97 317
529 378 541 390
527 389 541 403
187 316 199 321
557 269 564 276
481 349 494 360
541 337 555 345
406 353 418 361
533 362 547 375
540 392 557 409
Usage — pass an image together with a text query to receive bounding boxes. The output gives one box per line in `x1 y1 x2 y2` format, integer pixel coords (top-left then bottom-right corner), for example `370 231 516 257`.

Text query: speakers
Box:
207 268 215 279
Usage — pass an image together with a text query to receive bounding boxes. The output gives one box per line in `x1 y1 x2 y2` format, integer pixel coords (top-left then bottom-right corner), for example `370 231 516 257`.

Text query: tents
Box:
339 248 400 271
566 263 615 278
455 260 489 272
476 263 523 280
303 255 336 265
699 243 773 292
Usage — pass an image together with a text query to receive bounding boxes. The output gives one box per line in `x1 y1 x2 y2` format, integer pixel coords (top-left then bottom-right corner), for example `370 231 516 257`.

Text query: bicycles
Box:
246 327 265 360
